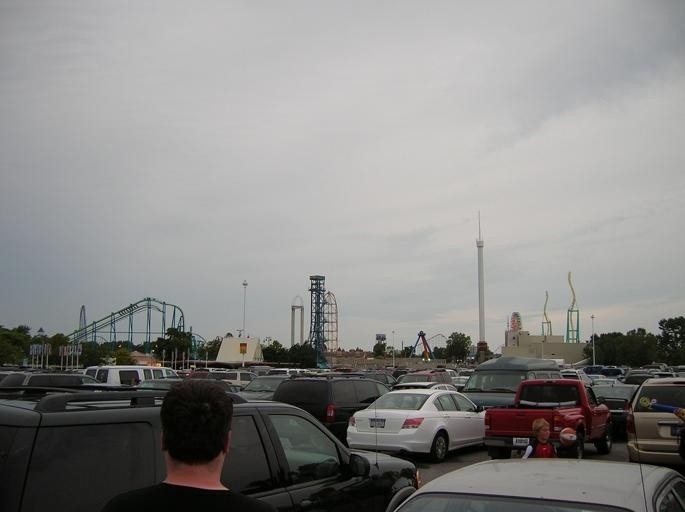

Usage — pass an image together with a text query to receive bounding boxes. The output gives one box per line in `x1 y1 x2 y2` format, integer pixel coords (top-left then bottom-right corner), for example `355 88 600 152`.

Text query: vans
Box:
461 357 566 410
625 376 685 466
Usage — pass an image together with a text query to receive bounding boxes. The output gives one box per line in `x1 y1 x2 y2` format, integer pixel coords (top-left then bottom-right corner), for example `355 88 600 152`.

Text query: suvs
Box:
272 373 393 447
0 383 422 512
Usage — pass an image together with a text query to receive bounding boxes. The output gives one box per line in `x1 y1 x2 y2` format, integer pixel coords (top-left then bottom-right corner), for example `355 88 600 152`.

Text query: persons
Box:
101 381 280 512
132 376 141 385
523 418 579 458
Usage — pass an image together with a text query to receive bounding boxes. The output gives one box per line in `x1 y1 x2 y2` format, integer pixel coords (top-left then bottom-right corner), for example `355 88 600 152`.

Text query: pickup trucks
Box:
482 378 615 459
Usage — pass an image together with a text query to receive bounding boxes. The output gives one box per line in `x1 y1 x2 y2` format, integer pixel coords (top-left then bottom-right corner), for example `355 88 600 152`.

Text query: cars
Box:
346 388 488 464
388 456 685 512
